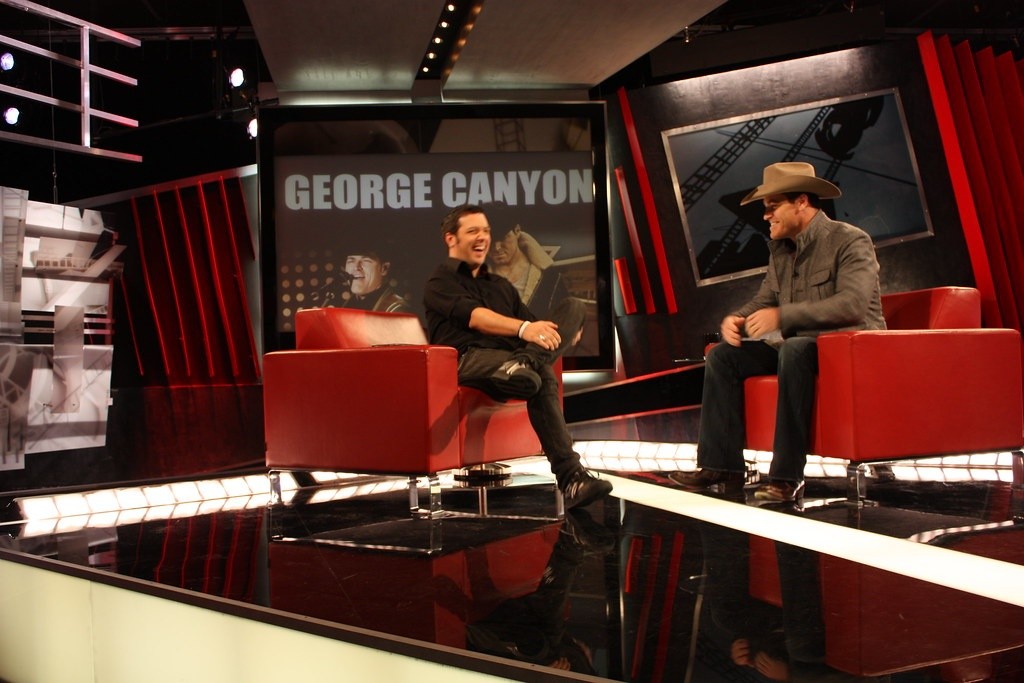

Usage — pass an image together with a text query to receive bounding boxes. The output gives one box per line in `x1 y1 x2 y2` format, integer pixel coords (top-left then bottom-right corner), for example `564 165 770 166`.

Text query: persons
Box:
342 246 416 313
484 199 571 320
422 205 611 512
468 485 827 683
668 162 889 509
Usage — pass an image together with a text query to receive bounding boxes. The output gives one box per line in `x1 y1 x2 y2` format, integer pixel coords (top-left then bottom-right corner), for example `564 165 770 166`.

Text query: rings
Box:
541 336 546 340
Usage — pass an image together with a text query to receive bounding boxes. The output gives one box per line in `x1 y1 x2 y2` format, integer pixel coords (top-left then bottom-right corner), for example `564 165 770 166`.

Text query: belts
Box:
668 468 745 491
755 478 805 500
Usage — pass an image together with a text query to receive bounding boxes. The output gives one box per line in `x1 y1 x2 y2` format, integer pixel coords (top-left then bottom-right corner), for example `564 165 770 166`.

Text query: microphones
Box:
308 271 351 301
791 272 798 278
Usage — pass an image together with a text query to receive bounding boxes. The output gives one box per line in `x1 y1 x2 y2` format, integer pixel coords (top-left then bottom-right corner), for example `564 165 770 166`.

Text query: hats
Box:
345 226 396 261
482 202 518 242
740 162 841 206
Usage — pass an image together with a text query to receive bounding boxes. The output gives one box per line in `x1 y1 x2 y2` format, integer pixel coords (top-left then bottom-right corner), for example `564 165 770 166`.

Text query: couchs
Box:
704 287 1024 509
264 308 562 555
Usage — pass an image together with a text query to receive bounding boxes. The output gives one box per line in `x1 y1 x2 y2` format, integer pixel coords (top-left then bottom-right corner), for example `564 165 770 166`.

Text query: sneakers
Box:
563 468 613 512
563 508 613 551
486 358 541 401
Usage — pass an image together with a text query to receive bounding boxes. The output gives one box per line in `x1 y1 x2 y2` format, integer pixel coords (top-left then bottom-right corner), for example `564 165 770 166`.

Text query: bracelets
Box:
517 320 531 339
